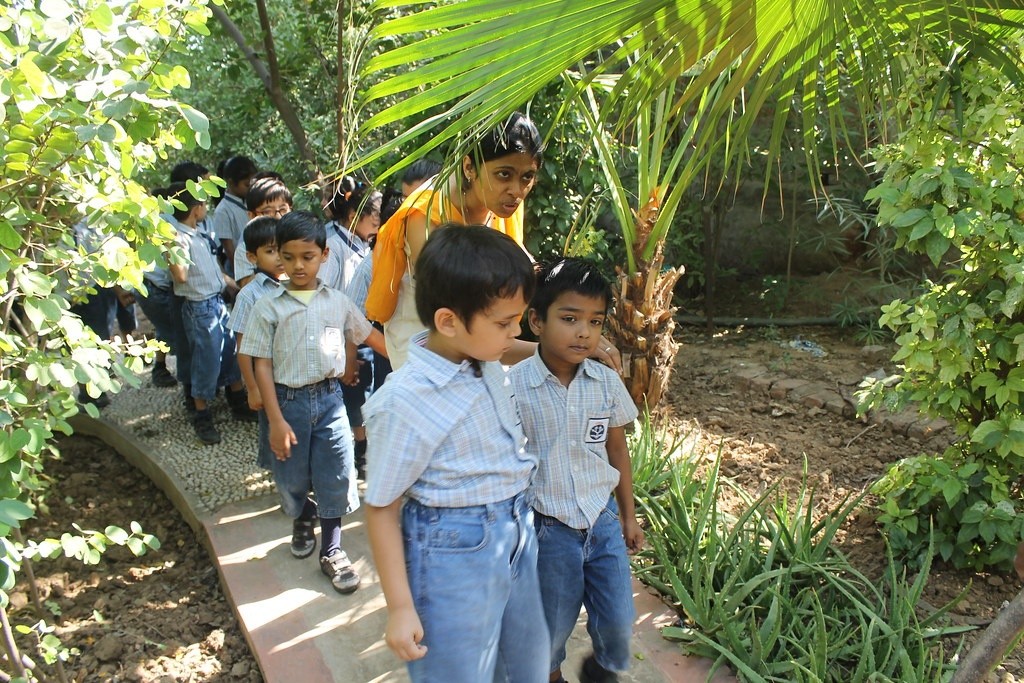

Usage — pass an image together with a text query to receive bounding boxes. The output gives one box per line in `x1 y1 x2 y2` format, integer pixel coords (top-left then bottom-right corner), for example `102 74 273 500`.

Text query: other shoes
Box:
354 454 366 478
152 368 177 387
78 392 110 407
225 386 232 406
579 660 620 683
184 396 195 410
232 401 259 422
215 388 220 396
192 412 220 444
124 353 153 367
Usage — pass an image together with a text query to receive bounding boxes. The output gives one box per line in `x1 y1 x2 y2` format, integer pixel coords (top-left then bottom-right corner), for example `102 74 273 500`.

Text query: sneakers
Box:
291 499 317 559
319 549 360 593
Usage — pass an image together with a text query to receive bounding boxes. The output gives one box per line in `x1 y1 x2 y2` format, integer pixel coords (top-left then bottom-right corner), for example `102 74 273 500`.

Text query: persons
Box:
212 156 258 278
54 161 258 443
317 175 393 478
365 111 624 371
401 159 443 201
233 177 294 287
224 215 286 470
359 222 551 683
239 209 389 593
504 256 644 683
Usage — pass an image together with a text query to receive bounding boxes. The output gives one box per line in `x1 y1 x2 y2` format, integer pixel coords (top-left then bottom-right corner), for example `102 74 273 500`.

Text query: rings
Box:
605 347 610 353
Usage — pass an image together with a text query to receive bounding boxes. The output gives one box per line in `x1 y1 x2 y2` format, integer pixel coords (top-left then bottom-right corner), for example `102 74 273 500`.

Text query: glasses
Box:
398 198 405 205
249 207 293 218
